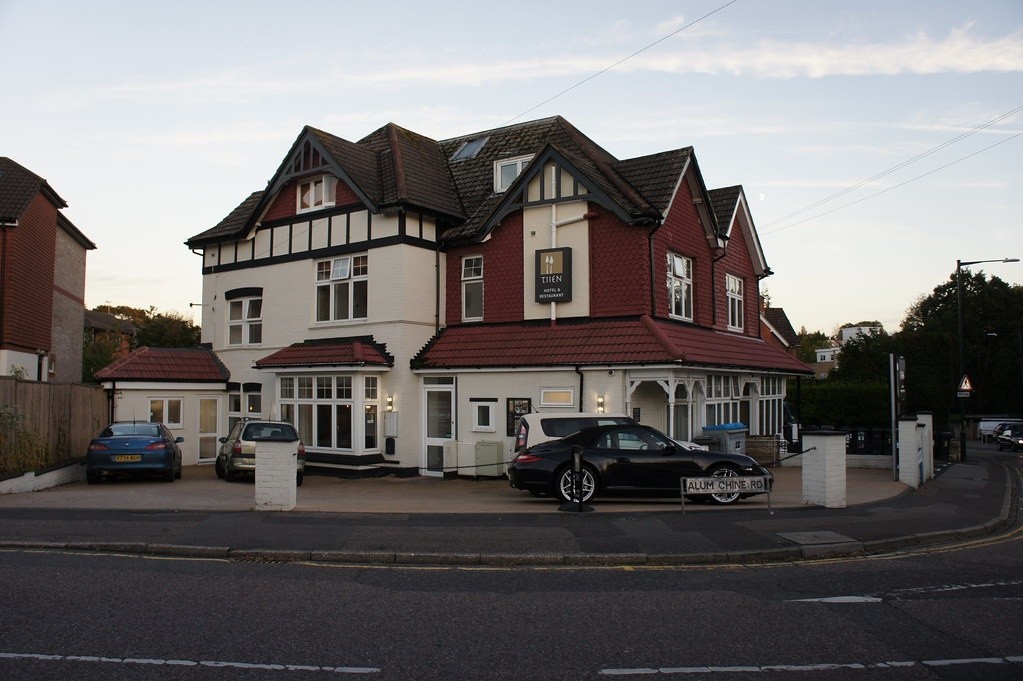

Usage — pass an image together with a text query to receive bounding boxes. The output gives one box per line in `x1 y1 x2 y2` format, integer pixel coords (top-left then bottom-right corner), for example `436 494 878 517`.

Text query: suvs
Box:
215 417 305 485
506 412 705 475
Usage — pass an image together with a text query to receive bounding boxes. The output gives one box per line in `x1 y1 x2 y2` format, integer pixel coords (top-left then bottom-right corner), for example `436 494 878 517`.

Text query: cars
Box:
992 422 1023 452
85 420 184 485
507 424 774 505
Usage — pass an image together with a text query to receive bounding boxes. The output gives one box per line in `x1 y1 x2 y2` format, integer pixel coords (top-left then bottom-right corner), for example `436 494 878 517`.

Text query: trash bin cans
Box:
691 436 721 452
702 422 748 455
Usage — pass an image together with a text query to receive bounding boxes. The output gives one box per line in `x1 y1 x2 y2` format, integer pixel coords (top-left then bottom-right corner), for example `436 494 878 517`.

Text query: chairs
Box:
246 431 253 441
271 430 281 435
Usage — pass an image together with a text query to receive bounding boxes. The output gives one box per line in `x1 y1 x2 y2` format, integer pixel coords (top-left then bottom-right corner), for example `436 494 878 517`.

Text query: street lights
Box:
956 258 1020 465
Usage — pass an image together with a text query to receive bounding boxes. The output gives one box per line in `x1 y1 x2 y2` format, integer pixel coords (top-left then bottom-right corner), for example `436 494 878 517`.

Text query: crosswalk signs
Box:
958 376 973 392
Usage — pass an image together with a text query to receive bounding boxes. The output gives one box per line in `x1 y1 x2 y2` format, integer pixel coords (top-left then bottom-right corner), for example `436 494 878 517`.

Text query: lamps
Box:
597 396 603 414
366 397 371 409
386 396 393 412
